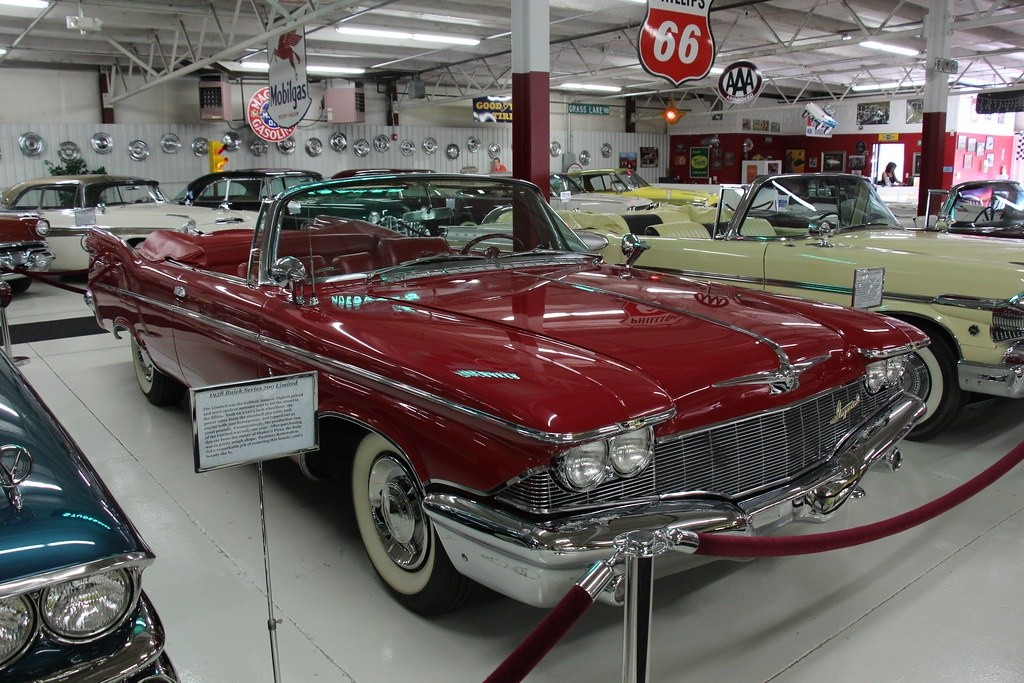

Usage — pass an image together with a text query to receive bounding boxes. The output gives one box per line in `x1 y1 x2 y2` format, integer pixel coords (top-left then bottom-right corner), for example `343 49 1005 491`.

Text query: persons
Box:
882 162 907 186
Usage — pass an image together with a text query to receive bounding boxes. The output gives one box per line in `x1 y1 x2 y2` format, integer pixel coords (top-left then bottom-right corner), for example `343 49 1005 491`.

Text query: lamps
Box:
238 59 365 75
840 34 923 56
561 82 628 93
335 22 481 45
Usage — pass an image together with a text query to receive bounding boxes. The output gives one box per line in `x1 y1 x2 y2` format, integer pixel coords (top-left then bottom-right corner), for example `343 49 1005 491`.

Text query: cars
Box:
0 168 1024 443
83 172 935 613
0 349 184 683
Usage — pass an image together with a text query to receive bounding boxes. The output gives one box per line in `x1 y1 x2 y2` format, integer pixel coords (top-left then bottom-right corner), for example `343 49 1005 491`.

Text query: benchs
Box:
146 219 407 279
493 204 737 237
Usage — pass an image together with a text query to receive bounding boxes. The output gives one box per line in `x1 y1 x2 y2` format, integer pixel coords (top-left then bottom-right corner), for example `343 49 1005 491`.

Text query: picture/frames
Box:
821 151 846 173
912 152 921 177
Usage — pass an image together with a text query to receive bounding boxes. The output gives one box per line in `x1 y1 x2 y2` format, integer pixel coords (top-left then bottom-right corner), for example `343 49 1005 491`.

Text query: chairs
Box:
237 238 453 282
644 217 781 240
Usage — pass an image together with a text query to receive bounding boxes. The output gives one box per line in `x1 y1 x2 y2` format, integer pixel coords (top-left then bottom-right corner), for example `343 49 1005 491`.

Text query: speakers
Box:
409 80 425 99
